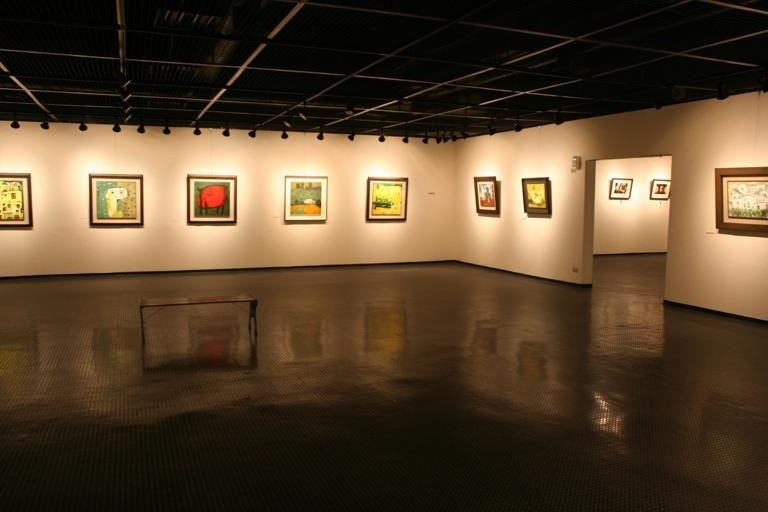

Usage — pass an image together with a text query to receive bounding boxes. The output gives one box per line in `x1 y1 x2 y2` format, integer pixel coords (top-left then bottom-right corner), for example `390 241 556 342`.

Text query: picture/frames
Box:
650 179 671 200
714 167 768 231
474 176 499 214
366 176 408 221
88 173 144 227
609 178 633 199
186 173 237 224
0 172 34 228
284 175 328 222
522 177 552 214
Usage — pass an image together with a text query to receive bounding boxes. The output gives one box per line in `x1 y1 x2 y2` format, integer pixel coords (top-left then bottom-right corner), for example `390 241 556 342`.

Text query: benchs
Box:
140 293 259 345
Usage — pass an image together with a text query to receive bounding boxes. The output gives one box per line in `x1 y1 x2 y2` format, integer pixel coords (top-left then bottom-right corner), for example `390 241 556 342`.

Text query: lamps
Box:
486 123 499 137
513 121 522 132
222 126 231 137
38 118 51 132
280 129 289 140
348 132 356 142
78 119 89 133
136 121 147 135
283 115 293 128
161 123 172 136
375 134 386 142
436 131 470 144
118 63 134 123
401 135 410 143
9 118 21 131
421 136 429 143
315 130 327 141
193 124 203 135
111 120 122 134
298 107 308 122
246 127 259 138
